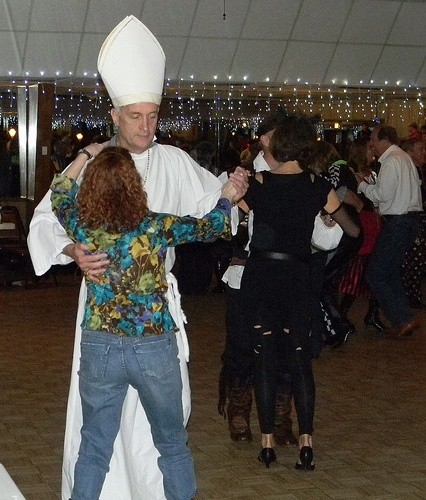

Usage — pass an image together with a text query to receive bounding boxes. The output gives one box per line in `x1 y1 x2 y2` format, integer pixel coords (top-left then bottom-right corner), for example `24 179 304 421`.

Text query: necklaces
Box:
142 148 150 187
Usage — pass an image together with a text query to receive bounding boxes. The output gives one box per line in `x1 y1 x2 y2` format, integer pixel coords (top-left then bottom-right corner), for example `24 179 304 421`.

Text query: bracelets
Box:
77 148 93 160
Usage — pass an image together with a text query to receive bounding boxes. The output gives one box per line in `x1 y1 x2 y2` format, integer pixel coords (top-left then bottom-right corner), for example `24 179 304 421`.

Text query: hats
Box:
97 14 167 109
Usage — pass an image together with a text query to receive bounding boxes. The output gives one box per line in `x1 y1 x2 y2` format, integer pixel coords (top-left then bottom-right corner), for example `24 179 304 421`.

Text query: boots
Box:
274 371 299 448
218 353 256 442
364 299 392 334
212 258 226 294
338 294 356 317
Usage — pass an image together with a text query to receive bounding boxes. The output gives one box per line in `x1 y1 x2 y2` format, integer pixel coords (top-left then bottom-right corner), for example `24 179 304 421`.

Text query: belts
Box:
251 248 305 261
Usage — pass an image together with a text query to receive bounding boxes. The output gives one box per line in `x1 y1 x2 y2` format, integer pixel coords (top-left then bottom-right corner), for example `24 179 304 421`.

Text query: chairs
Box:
0 206 60 287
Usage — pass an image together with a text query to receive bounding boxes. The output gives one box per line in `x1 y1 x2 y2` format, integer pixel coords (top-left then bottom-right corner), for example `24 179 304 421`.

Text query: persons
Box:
26 15 250 500
216 115 426 470
2 117 379 195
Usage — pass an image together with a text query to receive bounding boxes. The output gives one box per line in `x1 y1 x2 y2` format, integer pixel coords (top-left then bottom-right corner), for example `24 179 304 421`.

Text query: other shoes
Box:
327 325 356 347
392 319 420 339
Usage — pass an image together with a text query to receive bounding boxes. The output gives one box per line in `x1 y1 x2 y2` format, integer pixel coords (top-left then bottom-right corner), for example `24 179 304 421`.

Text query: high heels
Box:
258 448 277 468
295 446 315 470
